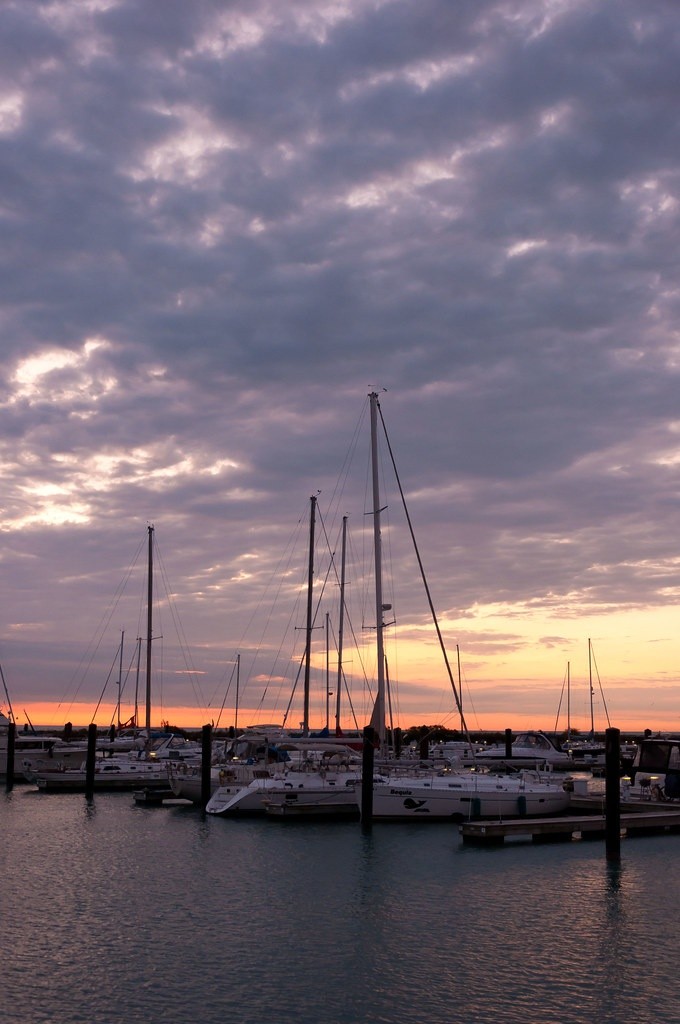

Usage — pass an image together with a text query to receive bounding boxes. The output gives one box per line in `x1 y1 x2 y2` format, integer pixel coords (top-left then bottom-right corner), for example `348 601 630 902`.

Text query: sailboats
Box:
0 386 680 829
